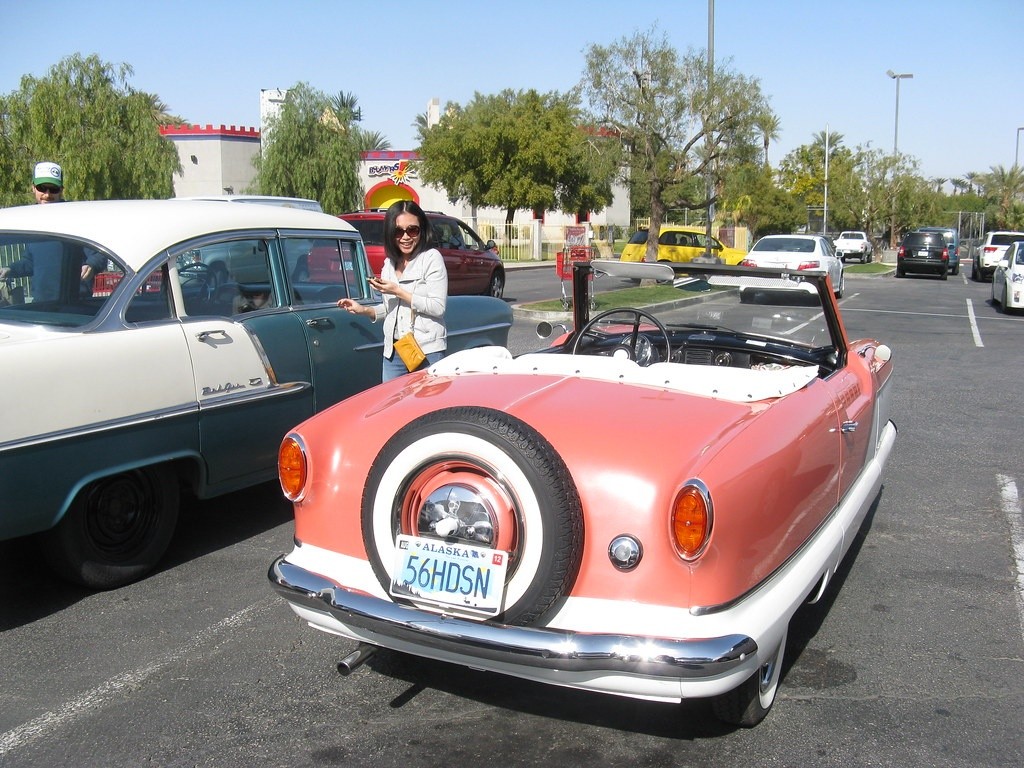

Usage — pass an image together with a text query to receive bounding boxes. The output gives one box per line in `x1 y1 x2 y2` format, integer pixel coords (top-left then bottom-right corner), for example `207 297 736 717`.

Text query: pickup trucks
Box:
833 231 873 263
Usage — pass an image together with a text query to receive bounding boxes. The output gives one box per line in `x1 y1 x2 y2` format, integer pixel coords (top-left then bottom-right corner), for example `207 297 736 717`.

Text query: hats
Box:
31 161 63 186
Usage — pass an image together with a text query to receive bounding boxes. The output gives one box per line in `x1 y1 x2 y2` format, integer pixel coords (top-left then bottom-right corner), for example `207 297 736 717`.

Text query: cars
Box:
619 224 747 284
990 240 1024 314
0 197 514 594
265 258 896 727
737 233 845 300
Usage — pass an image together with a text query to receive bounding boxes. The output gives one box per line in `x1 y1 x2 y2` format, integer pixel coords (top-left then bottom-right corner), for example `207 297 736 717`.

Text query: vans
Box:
308 207 506 304
148 196 324 295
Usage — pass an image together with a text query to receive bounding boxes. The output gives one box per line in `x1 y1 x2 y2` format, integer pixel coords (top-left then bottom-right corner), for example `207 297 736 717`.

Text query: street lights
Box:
885 69 913 248
1015 127 1024 170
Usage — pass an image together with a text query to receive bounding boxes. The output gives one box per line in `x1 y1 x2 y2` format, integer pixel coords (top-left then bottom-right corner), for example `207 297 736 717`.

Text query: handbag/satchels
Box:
393 309 426 372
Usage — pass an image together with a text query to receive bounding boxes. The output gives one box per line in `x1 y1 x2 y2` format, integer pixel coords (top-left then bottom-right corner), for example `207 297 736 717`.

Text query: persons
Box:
678 237 688 245
0 162 106 304
337 200 448 384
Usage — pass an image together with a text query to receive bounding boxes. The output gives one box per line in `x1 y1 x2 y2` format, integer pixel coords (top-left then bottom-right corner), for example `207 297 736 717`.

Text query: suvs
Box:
917 227 965 275
895 231 954 280
971 230 1024 282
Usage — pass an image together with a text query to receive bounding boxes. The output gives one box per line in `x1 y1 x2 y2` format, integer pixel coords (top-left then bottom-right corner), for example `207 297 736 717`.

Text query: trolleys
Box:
557 247 598 312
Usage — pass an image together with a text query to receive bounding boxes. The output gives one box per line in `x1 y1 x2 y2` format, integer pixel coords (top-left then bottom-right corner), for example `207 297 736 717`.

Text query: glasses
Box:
36 184 60 194
394 225 421 238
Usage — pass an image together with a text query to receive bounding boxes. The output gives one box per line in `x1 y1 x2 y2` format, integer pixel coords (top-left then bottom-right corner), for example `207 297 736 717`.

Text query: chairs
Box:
667 236 677 245
432 226 446 243
680 237 688 245
